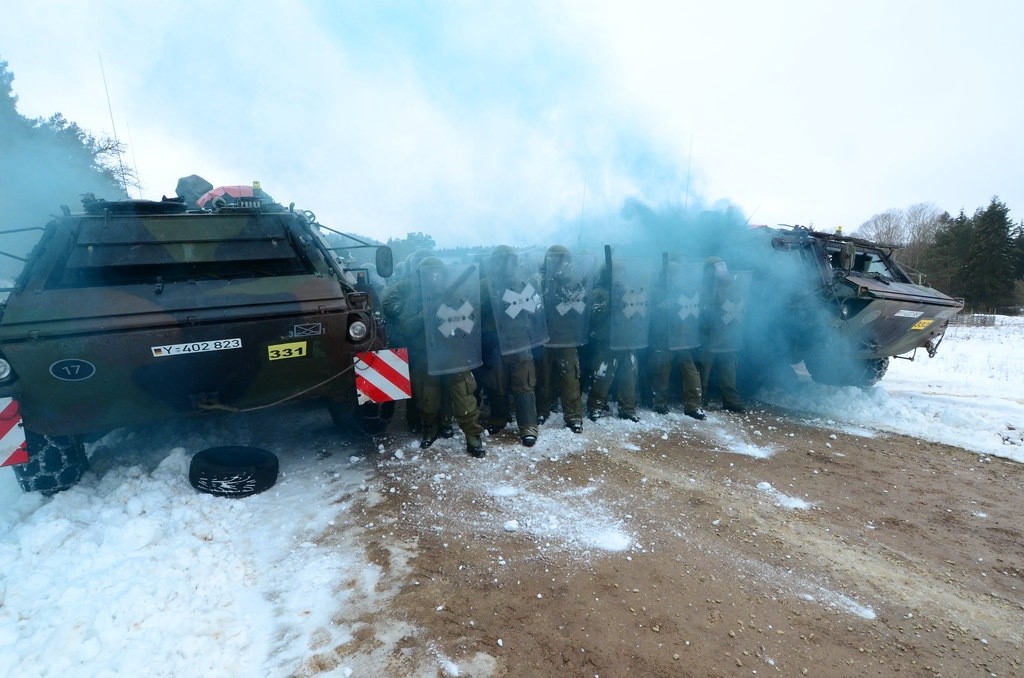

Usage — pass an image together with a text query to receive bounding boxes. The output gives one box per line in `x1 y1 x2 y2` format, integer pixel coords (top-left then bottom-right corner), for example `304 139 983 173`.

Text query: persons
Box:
361 239 750 457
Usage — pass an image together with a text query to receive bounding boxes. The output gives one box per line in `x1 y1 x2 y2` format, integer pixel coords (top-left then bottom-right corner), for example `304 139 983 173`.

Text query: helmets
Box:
394 247 446 286
486 245 516 260
546 245 571 256
595 261 626 285
703 255 724 267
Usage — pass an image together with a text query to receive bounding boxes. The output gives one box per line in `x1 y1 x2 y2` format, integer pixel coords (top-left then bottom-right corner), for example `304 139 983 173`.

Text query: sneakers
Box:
567 420 584 432
618 411 641 422
501 414 513 429
684 406 708 420
648 399 670 415
489 424 505 434
466 444 486 458
537 415 547 425
702 399 708 407
589 408 602 422
551 405 559 413
603 404 610 411
420 433 439 449
441 425 454 438
522 435 537 446
722 401 747 414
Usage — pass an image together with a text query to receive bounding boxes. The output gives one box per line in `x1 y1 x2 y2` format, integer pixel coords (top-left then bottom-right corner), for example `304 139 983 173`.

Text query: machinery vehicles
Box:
686 218 966 390
0 173 395 498
